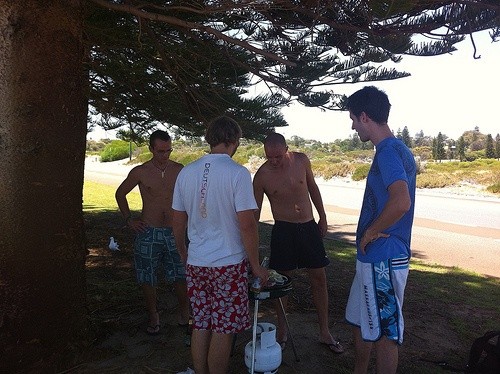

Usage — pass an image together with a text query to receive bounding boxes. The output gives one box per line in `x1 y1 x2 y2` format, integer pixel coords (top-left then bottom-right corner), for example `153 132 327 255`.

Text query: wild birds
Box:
109 236 121 252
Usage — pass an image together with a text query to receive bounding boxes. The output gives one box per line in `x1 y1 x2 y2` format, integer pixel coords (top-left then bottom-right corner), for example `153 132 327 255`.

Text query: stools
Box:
230 274 300 374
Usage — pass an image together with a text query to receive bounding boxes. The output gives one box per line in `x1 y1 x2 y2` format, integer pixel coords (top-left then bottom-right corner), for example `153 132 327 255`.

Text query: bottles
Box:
250 256 271 293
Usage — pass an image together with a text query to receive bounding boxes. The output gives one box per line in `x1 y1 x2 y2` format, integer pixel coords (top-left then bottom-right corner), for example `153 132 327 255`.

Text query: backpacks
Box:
465 330 500 374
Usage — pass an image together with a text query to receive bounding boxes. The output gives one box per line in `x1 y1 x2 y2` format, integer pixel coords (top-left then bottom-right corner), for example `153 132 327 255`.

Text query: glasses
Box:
156 148 173 152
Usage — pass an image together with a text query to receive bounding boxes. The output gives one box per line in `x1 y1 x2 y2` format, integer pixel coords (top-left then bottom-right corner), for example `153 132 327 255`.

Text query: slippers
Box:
277 334 287 351
328 339 344 353
145 313 160 335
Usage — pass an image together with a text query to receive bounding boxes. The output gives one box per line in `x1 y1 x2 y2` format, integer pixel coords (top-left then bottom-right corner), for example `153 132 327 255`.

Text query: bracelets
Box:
125 213 134 223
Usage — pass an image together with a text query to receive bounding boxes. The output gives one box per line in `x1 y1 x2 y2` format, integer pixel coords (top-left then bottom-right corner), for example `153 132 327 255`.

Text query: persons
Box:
116 131 189 333
172 117 269 374
253 134 344 354
344 86 417 374
